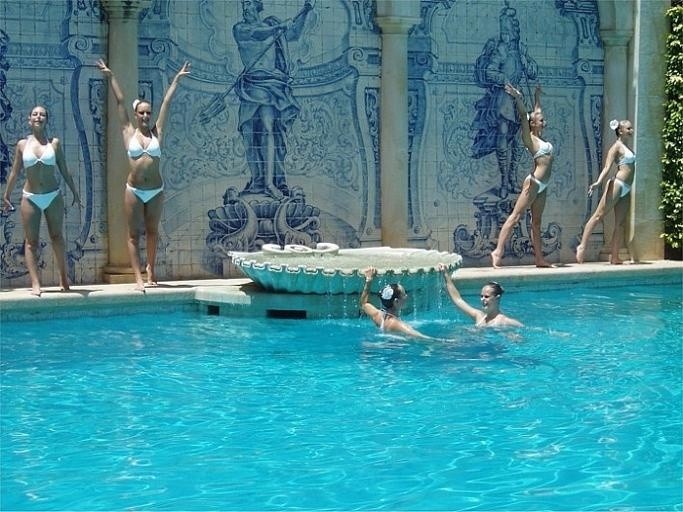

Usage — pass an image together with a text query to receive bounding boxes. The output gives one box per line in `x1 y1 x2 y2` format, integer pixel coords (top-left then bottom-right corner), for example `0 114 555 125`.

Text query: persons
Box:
473 6 541 201
92 55 192 292
2 103 82 298
575 119 637 265
488 82 554 269
356 267 452 344
433 263 575 345
225 1 314 201
